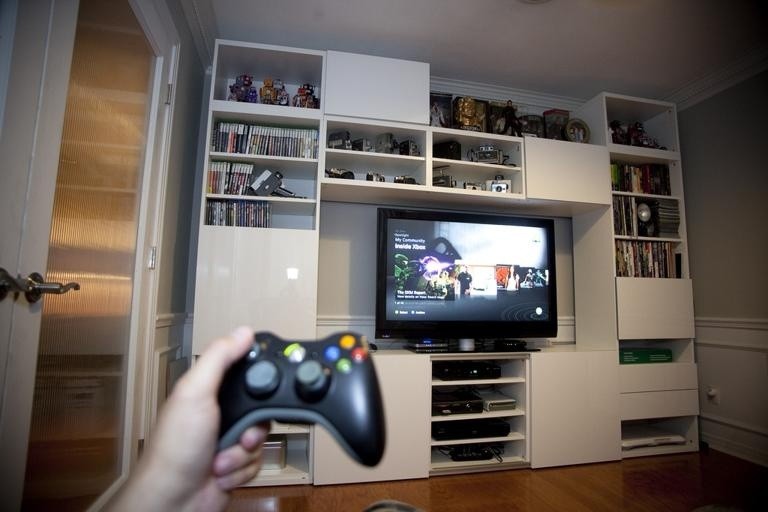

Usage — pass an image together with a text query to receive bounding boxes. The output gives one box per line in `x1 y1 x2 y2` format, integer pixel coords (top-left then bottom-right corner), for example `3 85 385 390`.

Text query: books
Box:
619 428 688 451
208 119 319 229
611 160 683 279
473 386 516 412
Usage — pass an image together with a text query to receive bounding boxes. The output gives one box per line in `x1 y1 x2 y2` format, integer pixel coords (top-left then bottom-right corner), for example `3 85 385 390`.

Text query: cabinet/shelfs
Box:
190 38 702 486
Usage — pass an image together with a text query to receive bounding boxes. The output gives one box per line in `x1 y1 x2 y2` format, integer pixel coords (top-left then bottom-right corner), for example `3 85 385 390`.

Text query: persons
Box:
457 264 473 300
503 265 520 292
498 97 522 139
455 98 480 124
98 326 271 512
523 267 535 290
534 268 547 288
430 102 442 126
446 265 460 301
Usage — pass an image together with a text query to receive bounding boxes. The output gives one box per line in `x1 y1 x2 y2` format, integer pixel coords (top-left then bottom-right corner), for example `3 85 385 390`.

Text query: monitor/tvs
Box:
375 208 558 354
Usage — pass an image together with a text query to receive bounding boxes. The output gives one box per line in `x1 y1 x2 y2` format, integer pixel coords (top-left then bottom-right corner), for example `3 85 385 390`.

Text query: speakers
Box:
634 198 658 237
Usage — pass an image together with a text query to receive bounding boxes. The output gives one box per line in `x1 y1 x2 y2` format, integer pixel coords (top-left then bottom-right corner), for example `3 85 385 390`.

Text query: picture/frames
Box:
565 118 590 143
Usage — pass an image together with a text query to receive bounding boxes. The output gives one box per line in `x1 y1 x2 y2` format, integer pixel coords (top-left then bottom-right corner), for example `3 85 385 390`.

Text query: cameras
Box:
471 146 503 164
399 136 420 156
375 133 399 154
486 175 512 193
394 175 421 185
433 165 457 187
248 169 296 197
328 131 352 150
366 169 385 182
464 181 482 191
352 138 372 152
433 141 461 160
326 167 354 180
